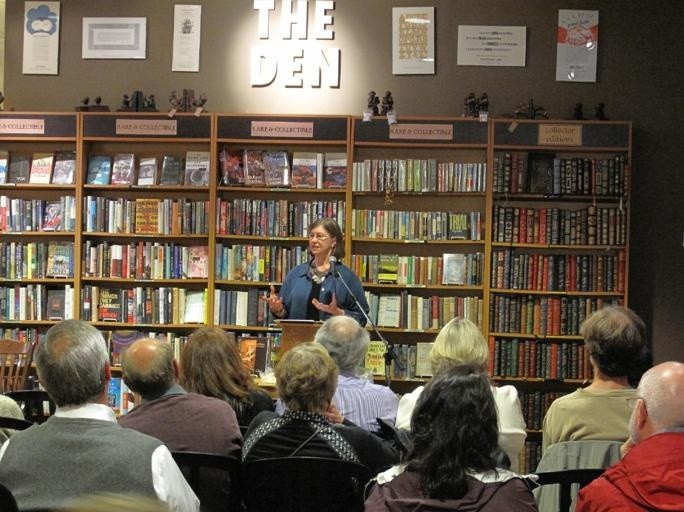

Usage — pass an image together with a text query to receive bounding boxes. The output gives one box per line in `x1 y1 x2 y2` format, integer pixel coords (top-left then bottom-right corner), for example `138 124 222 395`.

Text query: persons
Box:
260 218 371 328
571 362 683 512
118 315 526 468
0 319 201 512
364 365 540 512
542 304 649 459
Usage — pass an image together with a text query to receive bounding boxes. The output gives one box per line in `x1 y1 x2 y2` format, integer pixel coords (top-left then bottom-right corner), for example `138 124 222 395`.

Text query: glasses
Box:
626 397 648 416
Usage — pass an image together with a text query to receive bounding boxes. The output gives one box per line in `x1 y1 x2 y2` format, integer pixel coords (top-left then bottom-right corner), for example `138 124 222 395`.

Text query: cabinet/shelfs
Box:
210 114 352 385
0 110 80 417
77 112 215 418
488 118 633 432
348 115 491 383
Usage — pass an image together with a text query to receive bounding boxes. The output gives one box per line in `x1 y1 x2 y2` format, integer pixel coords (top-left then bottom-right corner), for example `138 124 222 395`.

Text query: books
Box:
78 152 208 415
1 150 76 359
351 160 487 373
494 153 628 428
211 149 347 376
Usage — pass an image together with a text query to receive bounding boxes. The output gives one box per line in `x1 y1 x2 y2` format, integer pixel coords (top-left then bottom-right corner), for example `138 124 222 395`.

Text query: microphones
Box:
327 255 337 279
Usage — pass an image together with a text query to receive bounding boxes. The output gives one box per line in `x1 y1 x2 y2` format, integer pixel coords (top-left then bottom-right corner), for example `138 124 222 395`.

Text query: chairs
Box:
0 340 37 407
0 384 628 512
